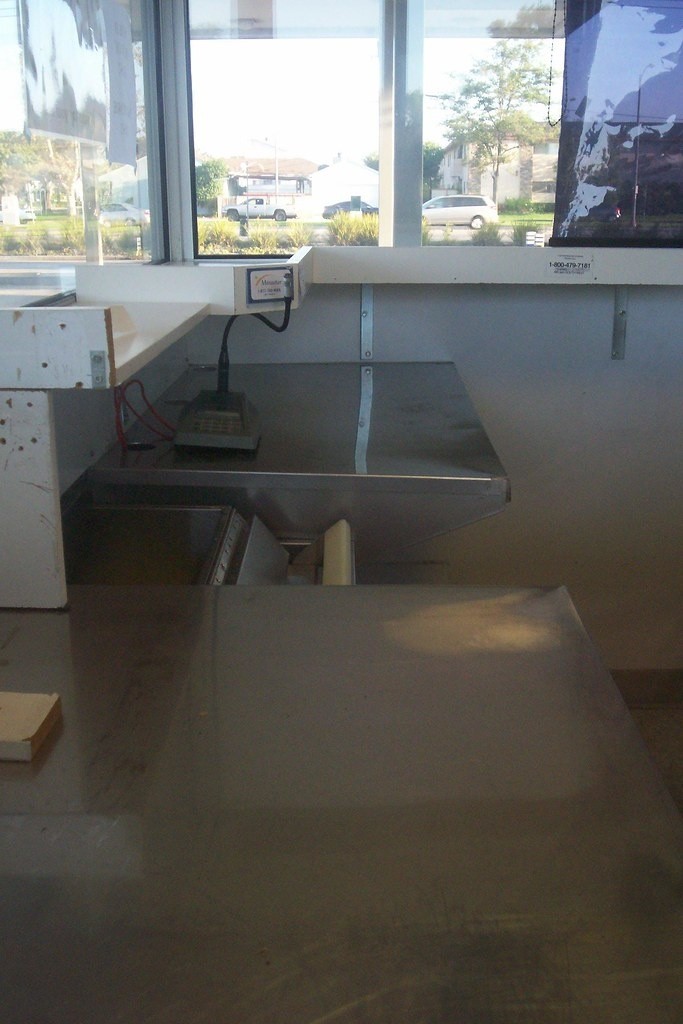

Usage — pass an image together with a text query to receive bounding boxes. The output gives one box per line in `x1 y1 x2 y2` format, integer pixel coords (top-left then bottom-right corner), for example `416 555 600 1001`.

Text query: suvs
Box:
422 193 497 230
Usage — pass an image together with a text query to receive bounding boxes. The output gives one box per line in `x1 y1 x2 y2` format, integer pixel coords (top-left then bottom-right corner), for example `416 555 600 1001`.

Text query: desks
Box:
0 585 683 1024
89 360 513 561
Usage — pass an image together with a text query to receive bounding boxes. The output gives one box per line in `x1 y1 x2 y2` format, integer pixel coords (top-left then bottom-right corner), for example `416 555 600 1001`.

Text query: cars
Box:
98 202 147 228
0 203 37 224
323 201 379 220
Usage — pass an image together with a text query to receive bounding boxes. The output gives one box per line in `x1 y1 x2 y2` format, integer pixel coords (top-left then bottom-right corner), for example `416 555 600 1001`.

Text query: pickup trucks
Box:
222 197 297 223
